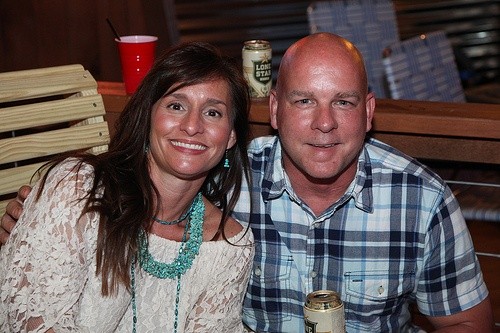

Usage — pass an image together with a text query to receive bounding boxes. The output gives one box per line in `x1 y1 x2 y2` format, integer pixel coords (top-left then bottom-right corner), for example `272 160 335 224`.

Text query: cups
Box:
114 35 159 96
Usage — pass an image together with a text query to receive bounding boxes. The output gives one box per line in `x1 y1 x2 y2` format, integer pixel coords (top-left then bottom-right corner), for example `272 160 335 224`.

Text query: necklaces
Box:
127 190 207 333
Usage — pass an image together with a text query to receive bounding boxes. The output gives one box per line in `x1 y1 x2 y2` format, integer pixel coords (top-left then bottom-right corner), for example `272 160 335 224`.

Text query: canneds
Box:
303 290 346 333
242 40 271 98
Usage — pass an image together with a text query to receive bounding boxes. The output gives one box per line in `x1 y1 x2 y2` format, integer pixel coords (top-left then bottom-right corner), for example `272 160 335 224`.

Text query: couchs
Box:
408 157 500 333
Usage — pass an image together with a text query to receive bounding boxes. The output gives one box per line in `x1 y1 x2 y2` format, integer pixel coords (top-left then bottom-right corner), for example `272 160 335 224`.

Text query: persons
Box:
0 41 258 333
1 31 493 333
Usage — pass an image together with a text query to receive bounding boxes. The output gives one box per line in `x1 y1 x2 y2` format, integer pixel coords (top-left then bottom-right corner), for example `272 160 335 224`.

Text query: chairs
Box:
0 65 111 240
